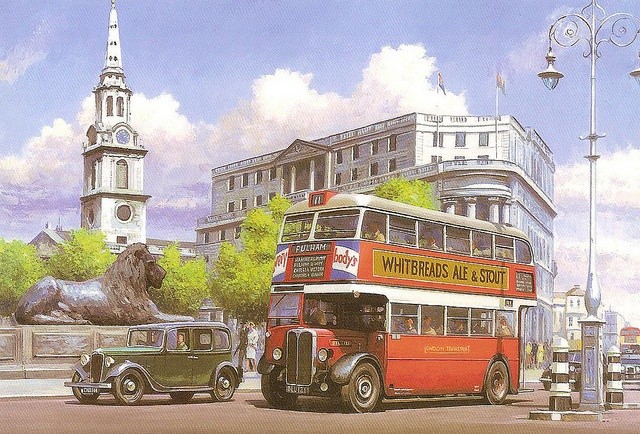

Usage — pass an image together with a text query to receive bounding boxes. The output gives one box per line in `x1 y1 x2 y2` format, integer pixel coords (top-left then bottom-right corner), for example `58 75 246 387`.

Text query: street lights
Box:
539 1 639 413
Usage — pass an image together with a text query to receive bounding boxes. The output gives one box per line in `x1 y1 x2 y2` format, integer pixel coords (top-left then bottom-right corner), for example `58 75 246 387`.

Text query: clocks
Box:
116 128 130 144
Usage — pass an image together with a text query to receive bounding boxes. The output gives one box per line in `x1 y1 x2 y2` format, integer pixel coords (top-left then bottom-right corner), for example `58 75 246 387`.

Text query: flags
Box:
497 75 506 95
439 72 446 95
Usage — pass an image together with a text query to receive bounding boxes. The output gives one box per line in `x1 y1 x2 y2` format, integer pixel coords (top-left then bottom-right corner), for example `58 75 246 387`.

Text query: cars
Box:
619 342 640 391
539 350 608 391
65 324 240 405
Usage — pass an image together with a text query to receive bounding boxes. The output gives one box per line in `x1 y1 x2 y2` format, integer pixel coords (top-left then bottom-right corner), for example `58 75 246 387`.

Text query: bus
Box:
256 190 539 414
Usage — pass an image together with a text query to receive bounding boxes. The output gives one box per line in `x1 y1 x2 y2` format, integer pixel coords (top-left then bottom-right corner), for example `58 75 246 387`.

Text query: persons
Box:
244 322 259 373
237 320 248 379
495 316 515 336
368 222 385 243
422 230 442 247
423 315 436 335
151 333 160 344
177 333 187 348
526 340 550 370
401 318 417 334
306 298 323 325
